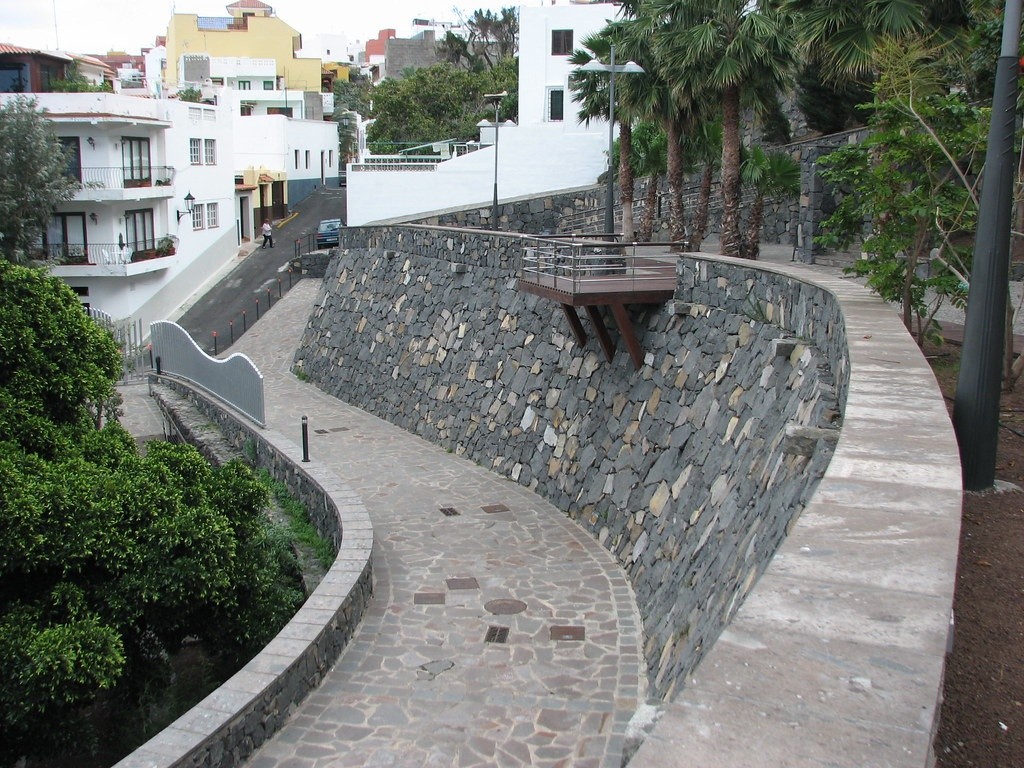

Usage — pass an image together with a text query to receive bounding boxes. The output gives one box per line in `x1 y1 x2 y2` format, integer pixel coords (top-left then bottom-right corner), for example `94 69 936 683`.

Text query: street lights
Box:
483 90 509 232
579 45 645 242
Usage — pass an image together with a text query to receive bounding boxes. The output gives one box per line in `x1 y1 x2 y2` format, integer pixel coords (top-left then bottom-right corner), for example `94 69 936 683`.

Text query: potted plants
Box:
130 237 174 262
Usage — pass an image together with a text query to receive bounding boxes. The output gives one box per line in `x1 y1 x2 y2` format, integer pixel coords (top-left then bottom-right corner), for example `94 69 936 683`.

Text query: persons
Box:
262 219 274 249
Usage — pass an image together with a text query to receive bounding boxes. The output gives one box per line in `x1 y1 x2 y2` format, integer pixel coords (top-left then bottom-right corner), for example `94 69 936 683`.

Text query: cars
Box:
315 218 347 250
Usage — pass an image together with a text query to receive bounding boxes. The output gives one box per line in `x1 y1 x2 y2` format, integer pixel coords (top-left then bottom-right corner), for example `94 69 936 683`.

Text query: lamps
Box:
176 190 195 225
88 212 97 225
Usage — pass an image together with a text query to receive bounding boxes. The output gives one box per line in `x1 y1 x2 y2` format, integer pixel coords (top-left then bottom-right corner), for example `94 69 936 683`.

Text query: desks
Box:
111 252 122 255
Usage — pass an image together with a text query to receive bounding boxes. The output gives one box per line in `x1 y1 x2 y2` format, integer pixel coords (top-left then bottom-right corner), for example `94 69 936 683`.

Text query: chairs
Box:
102 245 132 264
